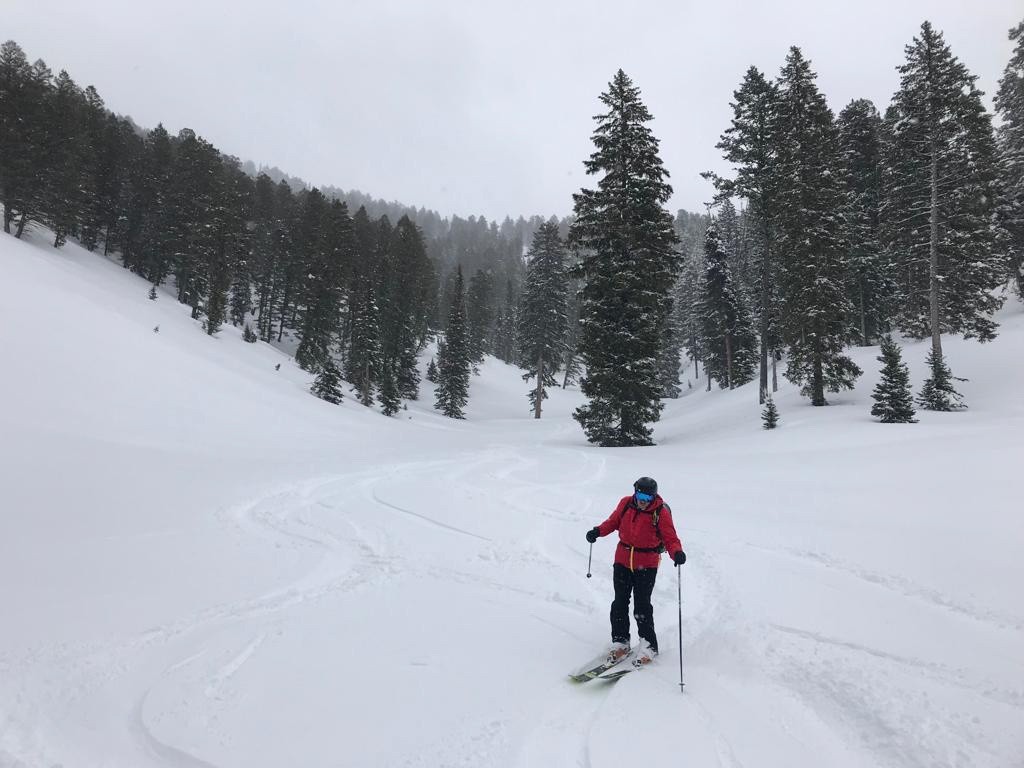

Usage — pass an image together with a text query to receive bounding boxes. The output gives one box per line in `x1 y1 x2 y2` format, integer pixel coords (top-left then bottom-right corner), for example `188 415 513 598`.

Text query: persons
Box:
585 477 686 667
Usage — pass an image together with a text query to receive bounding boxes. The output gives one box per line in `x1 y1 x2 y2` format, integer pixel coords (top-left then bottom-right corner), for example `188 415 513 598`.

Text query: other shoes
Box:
633 648 660 665
606 639 632 665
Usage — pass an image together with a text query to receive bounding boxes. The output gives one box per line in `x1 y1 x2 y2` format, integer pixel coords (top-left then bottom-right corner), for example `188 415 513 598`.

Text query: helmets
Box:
634 477 657 505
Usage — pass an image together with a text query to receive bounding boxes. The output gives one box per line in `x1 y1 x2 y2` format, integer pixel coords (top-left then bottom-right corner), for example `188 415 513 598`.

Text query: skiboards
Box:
567 646 660 682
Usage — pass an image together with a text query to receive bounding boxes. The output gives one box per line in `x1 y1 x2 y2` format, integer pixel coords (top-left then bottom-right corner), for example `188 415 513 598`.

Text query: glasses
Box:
635 490 654 503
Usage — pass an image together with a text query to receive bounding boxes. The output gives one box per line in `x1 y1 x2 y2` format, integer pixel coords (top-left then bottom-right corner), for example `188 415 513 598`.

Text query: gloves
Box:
586 527 599 543
674 551 686 567
